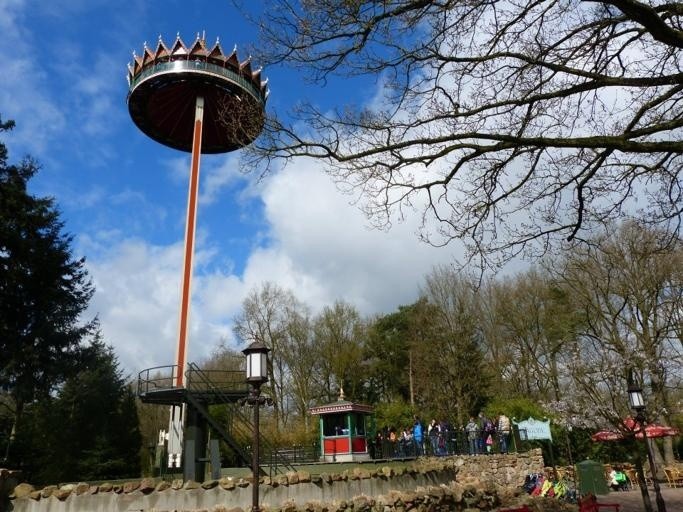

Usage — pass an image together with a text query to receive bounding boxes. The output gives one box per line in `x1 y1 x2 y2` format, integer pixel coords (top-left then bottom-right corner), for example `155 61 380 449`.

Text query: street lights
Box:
240 336 273 512
626 381 666 512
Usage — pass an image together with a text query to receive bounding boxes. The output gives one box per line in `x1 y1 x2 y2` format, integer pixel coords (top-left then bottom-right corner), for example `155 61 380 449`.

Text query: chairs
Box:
662 465 683 488
628 469 653 490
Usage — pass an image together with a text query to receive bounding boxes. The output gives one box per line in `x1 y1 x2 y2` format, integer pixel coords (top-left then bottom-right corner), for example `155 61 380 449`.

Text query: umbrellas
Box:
590 416 680 443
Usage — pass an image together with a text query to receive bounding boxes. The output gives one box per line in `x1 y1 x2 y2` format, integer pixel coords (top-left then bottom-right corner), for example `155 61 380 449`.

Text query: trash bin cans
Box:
578 459 609 495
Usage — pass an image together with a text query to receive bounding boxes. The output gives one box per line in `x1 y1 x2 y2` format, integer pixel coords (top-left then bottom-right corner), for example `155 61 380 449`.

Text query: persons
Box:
608 465 630 491
522 468 574 502
367 411 511 457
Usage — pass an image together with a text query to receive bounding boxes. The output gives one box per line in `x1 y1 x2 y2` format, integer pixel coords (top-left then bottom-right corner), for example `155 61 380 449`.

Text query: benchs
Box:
577 491 620 511
494 503 534 511
